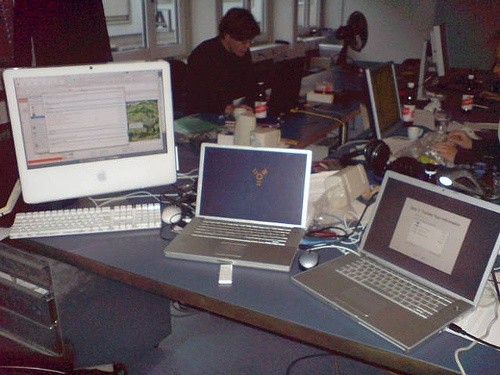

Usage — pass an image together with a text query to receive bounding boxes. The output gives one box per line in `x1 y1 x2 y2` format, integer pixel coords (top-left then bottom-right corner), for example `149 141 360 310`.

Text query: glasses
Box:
238 40 255 46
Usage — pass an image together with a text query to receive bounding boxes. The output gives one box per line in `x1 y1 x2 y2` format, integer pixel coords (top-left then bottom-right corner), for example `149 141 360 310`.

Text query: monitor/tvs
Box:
364 60 403 140
268 57 306 116
1 60 179 204
430 22 449 78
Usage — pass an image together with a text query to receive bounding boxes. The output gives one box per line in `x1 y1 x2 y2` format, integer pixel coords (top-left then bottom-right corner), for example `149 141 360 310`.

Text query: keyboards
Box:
409 130 459 166
9 202 161 239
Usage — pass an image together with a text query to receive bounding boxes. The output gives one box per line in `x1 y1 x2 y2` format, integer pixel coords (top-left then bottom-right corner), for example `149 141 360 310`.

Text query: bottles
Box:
461 75 475 114
402 83 416 126
437 114 448 143
421 163 438 185
254 81 266 123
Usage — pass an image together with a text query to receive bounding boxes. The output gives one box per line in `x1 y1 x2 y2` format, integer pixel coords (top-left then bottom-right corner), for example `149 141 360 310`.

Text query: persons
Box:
187 7 265 113
433 131 500 172
480 90 500 103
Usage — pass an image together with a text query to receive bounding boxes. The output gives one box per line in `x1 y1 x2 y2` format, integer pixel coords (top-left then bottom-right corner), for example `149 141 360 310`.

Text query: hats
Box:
220 8 260 38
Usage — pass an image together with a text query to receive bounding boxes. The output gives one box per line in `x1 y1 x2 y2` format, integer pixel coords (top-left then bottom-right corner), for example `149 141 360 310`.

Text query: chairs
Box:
166 58 187 119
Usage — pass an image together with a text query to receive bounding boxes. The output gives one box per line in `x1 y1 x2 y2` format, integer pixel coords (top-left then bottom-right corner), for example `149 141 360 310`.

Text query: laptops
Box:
164 142 312 272
289 170 500 354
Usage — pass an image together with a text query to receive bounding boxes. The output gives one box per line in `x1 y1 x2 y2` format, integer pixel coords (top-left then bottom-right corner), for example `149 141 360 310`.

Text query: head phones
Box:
332 139 389 169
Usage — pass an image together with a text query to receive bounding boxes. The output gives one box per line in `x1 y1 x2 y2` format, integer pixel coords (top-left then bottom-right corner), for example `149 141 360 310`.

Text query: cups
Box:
408 127 423 141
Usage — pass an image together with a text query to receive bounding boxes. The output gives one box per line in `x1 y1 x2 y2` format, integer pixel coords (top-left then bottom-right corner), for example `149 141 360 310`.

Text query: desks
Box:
0 59 499 373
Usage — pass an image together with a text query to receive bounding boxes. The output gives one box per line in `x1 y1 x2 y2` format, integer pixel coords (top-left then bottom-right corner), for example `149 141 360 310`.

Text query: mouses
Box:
298 251 320 271
161 206 181 224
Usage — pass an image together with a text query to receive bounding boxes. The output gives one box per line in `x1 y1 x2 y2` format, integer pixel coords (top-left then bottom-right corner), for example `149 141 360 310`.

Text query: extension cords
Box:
170 191 197 232
